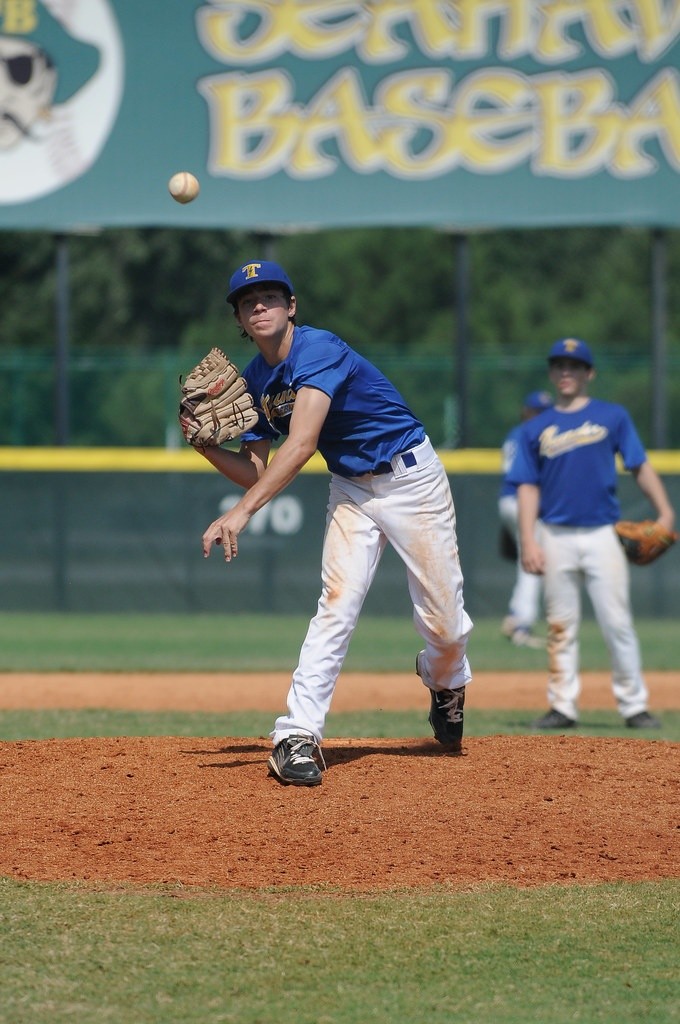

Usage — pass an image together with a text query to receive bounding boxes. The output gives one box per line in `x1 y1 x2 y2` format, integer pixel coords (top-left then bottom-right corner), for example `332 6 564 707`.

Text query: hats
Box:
546 336 594 366
524 389 552 409
225 259 295 304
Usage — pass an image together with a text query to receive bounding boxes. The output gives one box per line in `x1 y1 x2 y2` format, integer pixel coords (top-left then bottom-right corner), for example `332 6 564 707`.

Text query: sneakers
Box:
416 647 466 747
625 712 661 729
533 710 575 729
268 734 327 786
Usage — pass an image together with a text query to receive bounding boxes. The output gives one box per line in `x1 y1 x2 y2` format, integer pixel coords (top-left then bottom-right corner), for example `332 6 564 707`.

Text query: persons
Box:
178 260 474 787
496 337 677 729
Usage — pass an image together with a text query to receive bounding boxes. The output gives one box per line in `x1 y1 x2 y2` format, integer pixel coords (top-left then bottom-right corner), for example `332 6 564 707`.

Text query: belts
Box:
368 451 417 476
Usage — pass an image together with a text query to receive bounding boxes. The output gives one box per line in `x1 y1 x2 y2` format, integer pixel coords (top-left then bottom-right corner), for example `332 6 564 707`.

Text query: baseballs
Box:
168 171 201 204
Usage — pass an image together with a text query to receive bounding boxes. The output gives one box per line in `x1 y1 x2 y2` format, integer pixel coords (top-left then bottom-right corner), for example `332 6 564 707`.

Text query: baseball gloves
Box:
176 344 266 456
615 519 677 566
498 527 517 563
